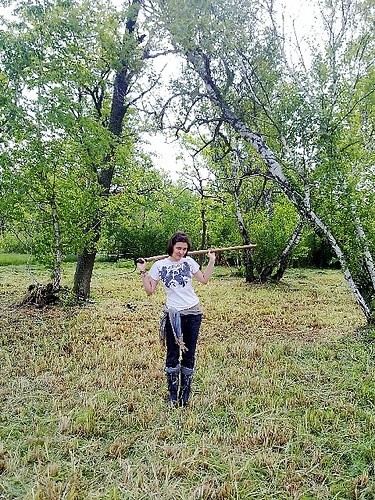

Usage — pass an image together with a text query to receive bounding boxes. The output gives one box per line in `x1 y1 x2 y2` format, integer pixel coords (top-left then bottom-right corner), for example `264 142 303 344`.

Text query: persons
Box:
137 232 216 407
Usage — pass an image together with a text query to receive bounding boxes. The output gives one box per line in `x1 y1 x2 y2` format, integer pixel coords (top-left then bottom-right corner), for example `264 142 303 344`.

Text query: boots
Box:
179 366 193 408
165 364 179 408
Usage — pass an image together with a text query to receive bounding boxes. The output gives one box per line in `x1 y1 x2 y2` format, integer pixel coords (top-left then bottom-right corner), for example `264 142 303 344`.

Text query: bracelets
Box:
139 270 146 276
